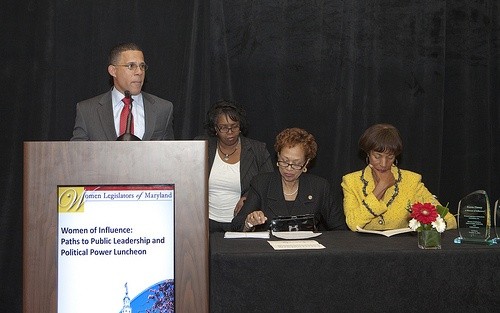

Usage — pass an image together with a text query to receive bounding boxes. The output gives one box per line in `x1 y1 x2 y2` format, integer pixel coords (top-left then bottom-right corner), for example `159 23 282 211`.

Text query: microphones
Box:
115 89 141 141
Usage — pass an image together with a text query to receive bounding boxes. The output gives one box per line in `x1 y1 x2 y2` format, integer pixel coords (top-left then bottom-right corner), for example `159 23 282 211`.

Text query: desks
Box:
210 228 500 313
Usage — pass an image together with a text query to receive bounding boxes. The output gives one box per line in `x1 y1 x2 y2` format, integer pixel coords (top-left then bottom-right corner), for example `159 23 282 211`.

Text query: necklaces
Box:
218 143 239 162
283 183 299 196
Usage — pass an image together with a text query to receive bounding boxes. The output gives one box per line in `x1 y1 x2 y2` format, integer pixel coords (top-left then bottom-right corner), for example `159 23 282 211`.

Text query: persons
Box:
340 124 458 231
189 99 273 233
71 42 174 141
230 129 347 231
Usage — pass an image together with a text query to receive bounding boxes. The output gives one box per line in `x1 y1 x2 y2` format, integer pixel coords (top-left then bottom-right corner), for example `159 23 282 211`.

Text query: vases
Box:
416 227 442 250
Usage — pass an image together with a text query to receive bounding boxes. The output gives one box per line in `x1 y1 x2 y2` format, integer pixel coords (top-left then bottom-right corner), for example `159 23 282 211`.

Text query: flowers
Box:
404 183 459 246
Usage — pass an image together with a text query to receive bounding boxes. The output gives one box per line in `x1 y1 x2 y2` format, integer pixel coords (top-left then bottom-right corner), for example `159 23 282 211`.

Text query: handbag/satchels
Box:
270 213 317 231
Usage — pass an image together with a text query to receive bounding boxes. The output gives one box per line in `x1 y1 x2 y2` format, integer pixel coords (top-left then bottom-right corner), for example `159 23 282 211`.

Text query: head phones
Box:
207 103 248 136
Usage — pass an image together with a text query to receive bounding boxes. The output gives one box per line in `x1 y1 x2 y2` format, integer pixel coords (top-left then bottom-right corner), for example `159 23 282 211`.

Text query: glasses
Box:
216 121 240 133
277 158 308 170
113 64 148 71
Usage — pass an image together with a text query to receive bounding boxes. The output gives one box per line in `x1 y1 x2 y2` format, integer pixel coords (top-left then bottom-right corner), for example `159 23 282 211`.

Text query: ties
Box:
120 95 134 136
